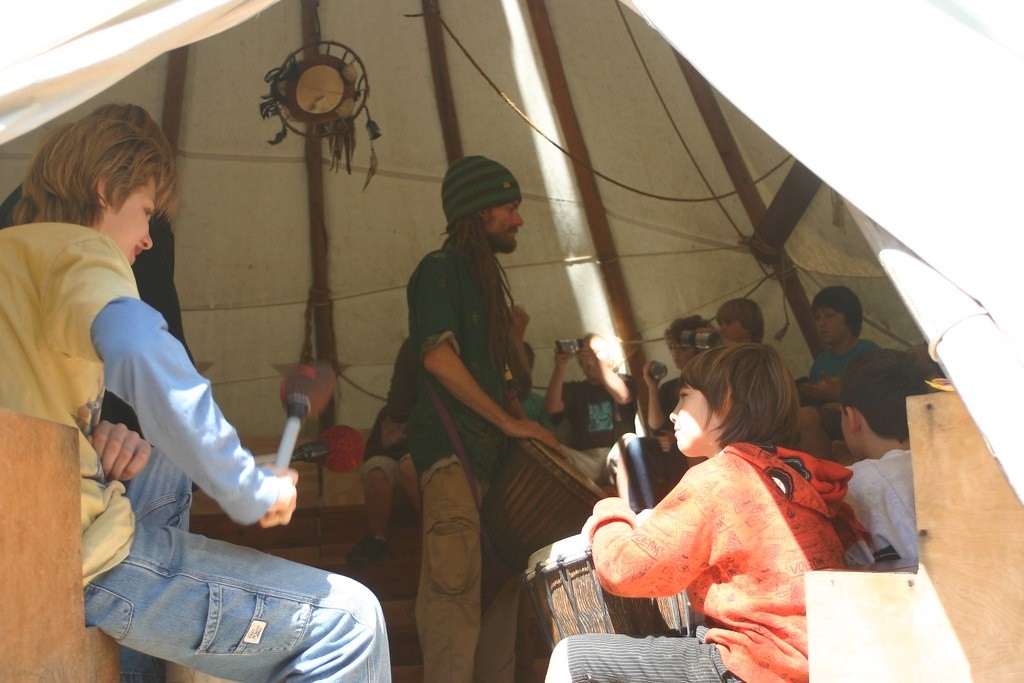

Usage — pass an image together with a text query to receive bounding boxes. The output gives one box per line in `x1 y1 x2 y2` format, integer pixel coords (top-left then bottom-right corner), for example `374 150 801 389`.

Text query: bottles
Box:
675 330 721 351
643 362 668 379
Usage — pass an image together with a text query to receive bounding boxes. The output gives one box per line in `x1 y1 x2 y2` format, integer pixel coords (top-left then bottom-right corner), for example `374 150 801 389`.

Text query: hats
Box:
441 155 522 222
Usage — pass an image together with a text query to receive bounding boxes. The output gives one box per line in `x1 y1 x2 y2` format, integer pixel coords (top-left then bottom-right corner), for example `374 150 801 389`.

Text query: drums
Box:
519 532 694 651
404 437 609 624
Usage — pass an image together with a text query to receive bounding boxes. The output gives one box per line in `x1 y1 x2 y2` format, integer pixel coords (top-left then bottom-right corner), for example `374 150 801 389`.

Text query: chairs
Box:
0 404 120 683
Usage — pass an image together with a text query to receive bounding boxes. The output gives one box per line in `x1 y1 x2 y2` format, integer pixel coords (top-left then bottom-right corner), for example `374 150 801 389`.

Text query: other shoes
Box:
342 533 390 565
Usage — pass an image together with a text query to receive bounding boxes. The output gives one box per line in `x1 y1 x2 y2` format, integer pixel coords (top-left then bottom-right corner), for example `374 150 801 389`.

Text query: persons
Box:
0 103 391 683
405 155 568 683
347 303 535 564
542 287 938 683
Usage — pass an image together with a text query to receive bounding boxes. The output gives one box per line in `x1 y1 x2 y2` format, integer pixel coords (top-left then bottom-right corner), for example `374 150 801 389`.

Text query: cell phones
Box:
556 339 582 357
